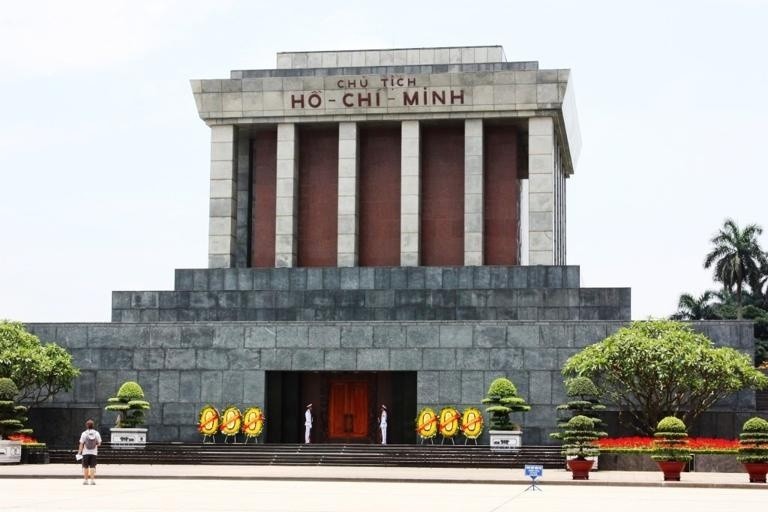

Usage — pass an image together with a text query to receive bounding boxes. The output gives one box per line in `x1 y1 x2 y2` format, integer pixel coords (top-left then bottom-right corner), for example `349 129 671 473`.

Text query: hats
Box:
382 405 387 409
306 404 312 408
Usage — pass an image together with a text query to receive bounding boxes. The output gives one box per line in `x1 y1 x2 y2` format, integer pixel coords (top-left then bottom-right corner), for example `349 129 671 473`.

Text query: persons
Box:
77 420 102 485
379 405 388 444
305 404 313 443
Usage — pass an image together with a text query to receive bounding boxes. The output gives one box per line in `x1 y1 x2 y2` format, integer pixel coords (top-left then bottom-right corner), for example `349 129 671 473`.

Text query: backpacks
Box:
85 433 97 449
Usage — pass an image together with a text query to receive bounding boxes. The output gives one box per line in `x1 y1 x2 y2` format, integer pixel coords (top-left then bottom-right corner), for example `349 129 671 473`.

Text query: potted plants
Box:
480 376 768 483
105 381 151 453
0 378 29 463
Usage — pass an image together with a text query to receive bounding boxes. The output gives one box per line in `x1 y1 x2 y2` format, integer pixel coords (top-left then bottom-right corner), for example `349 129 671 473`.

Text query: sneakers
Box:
91 481 95 485
83 481 88 485
